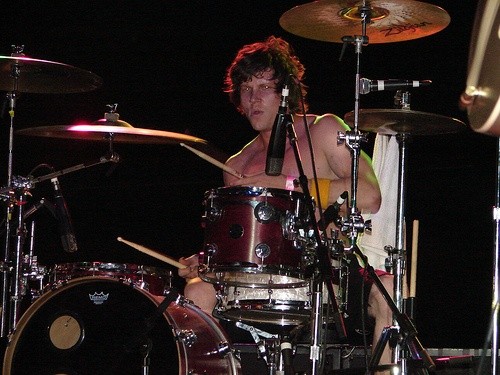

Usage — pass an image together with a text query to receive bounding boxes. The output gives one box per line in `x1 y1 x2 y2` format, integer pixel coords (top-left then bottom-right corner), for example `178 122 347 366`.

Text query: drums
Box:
198 185 317 290
2 276 243 375
43 262 174 294
212 256 345 333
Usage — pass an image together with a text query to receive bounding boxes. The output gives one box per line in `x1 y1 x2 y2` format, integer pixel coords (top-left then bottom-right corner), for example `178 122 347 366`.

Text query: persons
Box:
178 35 396 374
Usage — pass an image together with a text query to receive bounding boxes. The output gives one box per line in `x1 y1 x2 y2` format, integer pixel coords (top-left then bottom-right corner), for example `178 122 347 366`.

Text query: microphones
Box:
357 77 433 94
265 86 290 176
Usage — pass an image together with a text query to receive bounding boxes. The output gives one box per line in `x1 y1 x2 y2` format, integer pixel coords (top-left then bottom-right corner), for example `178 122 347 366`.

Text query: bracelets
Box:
310 177 330 211
285 174 296 191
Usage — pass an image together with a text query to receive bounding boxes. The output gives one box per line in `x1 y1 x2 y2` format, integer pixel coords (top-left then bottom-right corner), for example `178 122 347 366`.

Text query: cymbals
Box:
0 55 101 94
344 109 464 138
278 0 451 45
14 120 207 144
